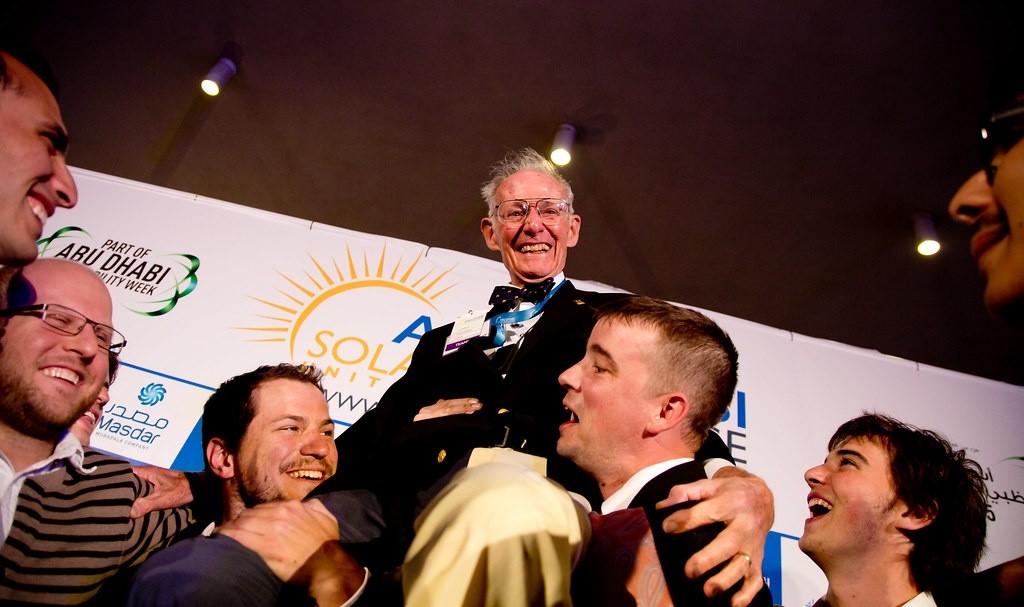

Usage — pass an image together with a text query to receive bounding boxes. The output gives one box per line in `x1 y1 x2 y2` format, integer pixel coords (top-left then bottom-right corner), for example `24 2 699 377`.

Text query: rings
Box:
736 550 753 570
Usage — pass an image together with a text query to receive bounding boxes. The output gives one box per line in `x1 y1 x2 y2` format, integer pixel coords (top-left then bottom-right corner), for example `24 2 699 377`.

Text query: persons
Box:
554 299 780 606
1 47 196 607
796 412 992 607
79 143 776 607
187 364 405 606
949 71 1023 325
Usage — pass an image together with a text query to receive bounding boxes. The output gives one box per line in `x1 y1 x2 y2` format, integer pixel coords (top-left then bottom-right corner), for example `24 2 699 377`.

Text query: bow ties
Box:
488 277 554 307
587 508 650 594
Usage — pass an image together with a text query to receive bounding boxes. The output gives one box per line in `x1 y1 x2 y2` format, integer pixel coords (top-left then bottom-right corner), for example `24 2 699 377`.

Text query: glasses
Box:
980 107 1024 152
0 303 127 356
490 197 570 227
